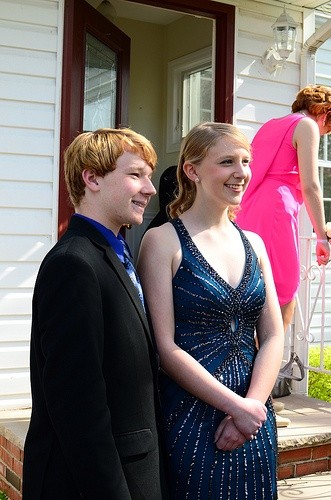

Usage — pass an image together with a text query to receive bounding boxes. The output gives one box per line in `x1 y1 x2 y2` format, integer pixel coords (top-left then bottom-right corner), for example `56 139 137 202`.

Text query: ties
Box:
120 240 146 316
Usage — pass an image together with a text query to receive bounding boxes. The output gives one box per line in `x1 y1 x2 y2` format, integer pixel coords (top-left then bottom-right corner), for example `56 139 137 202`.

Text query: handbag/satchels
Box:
272 352 305 399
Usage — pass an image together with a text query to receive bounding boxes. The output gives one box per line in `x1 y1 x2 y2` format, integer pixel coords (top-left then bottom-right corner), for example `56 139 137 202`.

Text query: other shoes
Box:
275 414 290 427
272 401 284 412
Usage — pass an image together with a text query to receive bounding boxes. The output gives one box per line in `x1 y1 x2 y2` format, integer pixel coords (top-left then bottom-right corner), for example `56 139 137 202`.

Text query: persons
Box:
21 126 162 500
231 84 331 427
136 122 286 500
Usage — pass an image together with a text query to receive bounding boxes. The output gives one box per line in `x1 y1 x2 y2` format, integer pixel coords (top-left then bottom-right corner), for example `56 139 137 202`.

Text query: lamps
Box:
272 2 297 61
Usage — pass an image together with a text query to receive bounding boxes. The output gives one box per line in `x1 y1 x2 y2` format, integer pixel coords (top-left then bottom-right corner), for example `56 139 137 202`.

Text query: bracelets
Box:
317 239 328 243
